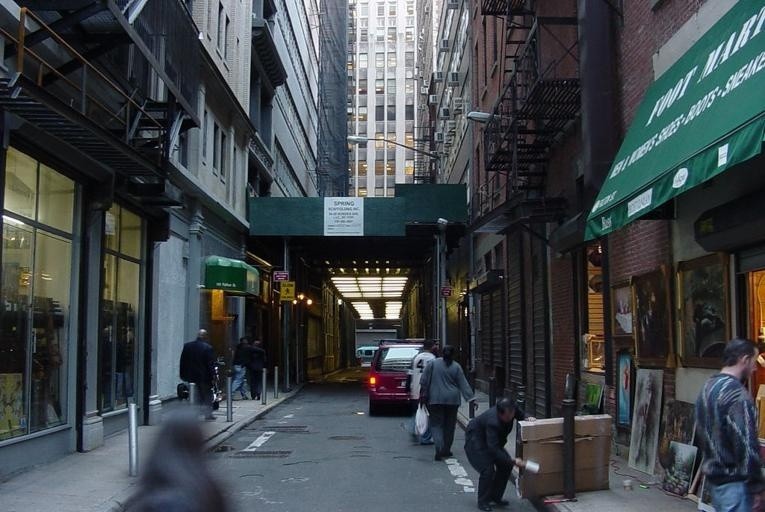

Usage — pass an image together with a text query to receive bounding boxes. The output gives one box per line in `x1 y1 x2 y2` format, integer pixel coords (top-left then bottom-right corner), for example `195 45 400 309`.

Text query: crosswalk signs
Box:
280 279 296 302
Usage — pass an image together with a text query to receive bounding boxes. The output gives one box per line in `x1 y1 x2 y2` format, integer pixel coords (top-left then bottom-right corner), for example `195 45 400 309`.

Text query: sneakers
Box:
435 450 453 461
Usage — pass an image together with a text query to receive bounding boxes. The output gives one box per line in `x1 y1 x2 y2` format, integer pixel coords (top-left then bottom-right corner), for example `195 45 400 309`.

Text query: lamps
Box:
466 110 529 126
346 135 441 164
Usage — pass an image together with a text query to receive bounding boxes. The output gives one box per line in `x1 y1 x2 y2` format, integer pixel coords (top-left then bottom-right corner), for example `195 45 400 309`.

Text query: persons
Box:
115 407 229 512
416 344 479 461
463 395 537 511
633 371 655 469
693 337 765 511
179 327 218 421
405 338 435 445
622 360 631 409
97 326 110 409
693 300 725 338
0 379 21 419
231 335 266 401
248 339 268 400
617 290 657 345
38 326 62 424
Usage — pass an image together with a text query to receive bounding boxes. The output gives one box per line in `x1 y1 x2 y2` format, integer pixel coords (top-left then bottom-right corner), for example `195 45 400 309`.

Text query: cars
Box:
368 341 427 416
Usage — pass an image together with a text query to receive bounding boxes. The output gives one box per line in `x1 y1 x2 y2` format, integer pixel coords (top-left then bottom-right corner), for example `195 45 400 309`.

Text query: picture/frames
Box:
610 253 732 370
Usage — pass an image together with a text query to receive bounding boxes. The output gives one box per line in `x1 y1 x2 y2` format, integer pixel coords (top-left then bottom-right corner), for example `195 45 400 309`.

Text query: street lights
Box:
346 134 445 357
468 110 528 416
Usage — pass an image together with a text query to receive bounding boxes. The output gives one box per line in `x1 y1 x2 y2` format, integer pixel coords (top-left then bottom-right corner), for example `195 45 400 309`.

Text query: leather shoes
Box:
478 500 509 511
